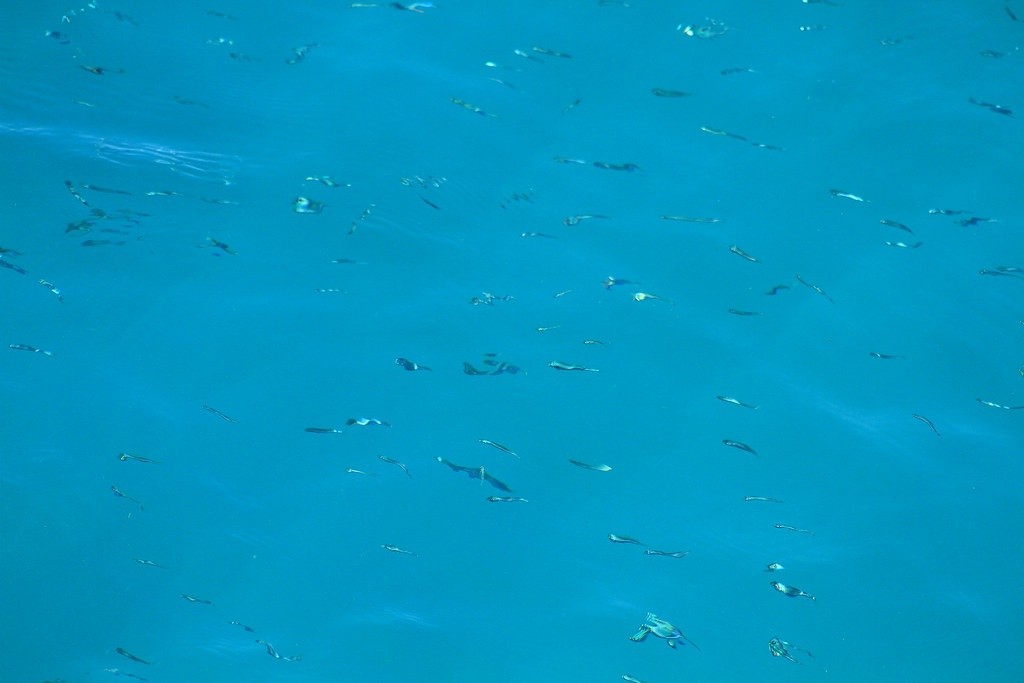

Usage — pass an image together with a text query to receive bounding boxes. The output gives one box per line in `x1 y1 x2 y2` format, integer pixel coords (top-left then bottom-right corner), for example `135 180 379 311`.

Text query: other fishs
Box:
1 0 1023 683
